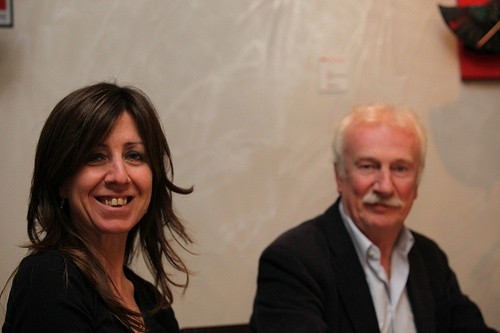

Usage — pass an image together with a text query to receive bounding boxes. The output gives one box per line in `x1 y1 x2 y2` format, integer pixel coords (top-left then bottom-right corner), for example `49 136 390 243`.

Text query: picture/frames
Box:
0 0 14 27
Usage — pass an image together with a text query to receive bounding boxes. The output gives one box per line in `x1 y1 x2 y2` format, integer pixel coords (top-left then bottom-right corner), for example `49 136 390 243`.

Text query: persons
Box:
0 82 196 333
250 104 500 333
